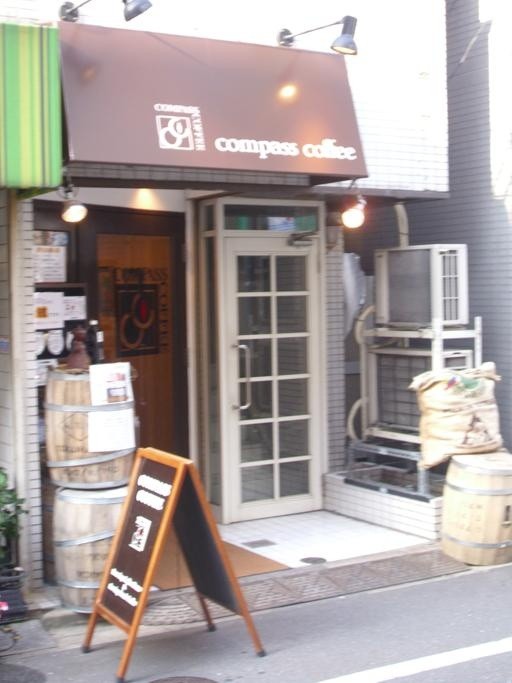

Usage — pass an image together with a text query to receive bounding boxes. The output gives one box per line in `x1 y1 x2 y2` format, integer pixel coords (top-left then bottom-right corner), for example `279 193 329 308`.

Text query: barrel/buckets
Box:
38 443 61 588
437 454 512 565
48 487 128 614
43 363 141 490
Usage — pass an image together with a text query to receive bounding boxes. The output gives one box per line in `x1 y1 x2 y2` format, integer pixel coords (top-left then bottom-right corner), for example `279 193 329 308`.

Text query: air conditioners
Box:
344 202 484 458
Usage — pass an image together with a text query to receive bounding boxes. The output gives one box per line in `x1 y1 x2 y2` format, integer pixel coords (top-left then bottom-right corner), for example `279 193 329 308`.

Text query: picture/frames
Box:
114 281 160 358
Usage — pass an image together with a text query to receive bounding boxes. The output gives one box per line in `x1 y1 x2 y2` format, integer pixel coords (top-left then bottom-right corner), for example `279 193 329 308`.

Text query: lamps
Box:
276 13 359 55
57 0 153 22
59 173 88 223
341 176 367 229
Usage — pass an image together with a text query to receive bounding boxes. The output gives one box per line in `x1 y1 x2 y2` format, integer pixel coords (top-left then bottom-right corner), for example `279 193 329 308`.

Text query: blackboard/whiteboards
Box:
93 447 188 636
